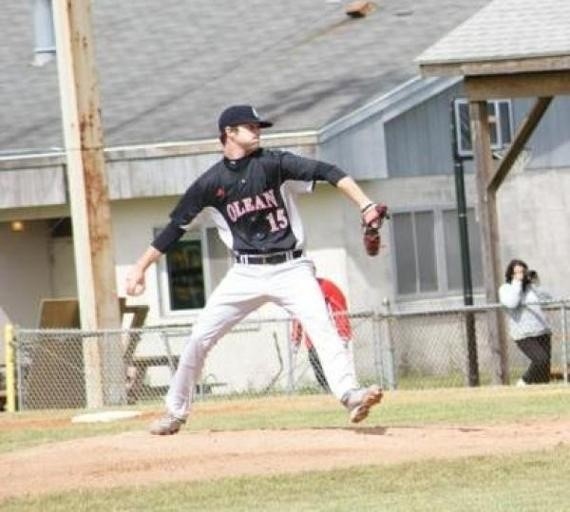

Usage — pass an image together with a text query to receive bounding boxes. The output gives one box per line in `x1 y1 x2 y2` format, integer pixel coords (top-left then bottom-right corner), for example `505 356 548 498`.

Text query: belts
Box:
234 248 303 265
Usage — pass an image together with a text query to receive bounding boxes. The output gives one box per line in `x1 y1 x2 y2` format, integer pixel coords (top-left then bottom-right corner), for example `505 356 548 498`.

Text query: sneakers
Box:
148 412 186 436
340 383 385 424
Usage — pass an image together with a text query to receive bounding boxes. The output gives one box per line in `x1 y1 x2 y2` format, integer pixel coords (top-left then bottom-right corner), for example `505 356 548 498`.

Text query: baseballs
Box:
134 284 144 295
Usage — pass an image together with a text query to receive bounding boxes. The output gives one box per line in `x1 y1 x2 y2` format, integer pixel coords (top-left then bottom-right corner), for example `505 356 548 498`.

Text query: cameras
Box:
520 266 538 286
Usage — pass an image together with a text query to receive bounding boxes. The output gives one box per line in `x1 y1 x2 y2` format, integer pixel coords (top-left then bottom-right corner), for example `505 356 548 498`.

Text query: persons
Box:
125 105 385 437
498 259 553 386
291 277 352 394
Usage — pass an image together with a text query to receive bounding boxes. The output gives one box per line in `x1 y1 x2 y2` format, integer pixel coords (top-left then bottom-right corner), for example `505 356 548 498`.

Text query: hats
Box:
218 105 274 130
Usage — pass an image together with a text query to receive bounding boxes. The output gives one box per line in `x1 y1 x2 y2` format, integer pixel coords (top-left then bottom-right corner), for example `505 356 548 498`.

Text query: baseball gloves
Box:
360 202 387 257
